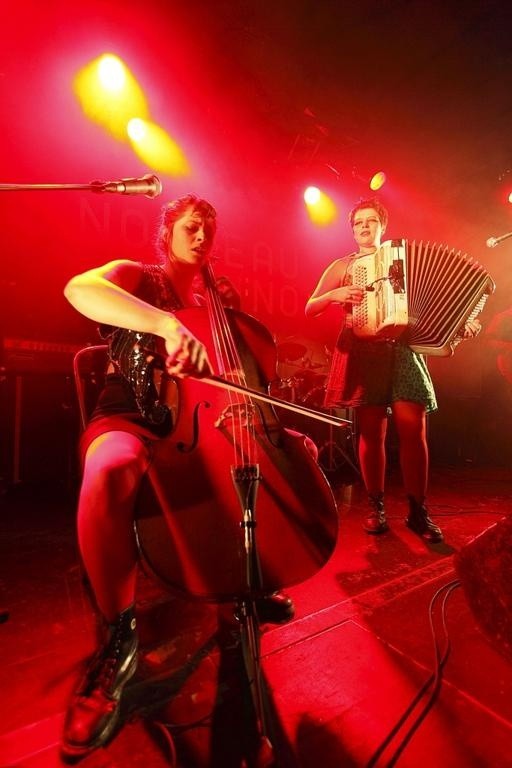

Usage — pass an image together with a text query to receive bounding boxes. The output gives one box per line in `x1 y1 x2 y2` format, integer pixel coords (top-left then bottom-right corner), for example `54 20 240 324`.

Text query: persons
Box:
306 198 446 544
57 196 340 755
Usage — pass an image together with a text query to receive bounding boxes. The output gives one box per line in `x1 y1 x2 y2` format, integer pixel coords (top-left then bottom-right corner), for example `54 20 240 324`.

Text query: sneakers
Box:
256 589 294 625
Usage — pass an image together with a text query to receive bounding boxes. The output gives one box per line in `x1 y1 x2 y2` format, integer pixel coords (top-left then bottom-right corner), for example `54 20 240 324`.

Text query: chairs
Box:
72 344 114 434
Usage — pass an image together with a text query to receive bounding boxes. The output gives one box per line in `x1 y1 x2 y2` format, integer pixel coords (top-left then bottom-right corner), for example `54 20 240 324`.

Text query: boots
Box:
404 493 443 543
362 491 387 533
60 603 139 756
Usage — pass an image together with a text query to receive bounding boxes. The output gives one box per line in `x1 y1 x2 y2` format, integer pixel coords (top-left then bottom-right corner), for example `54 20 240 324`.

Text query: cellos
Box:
125 260 337 601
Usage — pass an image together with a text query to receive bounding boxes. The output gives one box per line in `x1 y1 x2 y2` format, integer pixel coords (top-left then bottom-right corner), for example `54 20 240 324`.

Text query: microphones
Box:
484 231 512 249
99 173 162 201
